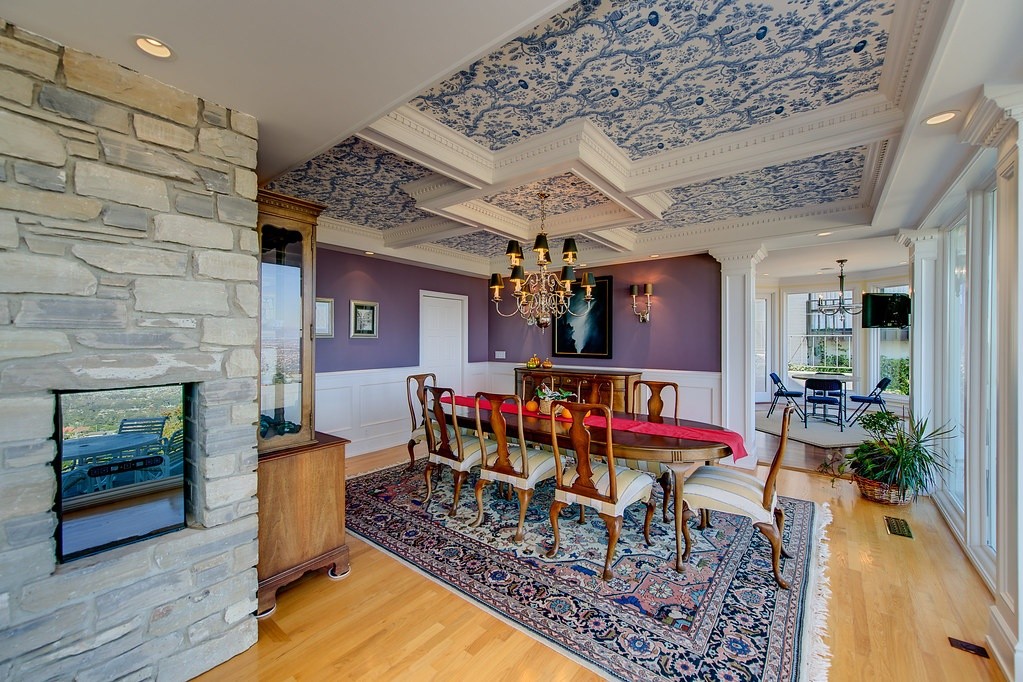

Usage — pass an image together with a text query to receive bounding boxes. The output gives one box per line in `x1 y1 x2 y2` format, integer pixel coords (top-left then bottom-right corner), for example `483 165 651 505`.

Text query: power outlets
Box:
495 351 506 359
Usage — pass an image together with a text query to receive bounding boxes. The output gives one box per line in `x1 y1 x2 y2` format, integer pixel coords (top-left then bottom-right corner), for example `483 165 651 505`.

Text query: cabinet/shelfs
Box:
256 187 353 623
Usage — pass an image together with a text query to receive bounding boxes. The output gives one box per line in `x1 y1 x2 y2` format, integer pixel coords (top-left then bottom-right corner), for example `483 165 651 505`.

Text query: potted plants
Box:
816 407 958 505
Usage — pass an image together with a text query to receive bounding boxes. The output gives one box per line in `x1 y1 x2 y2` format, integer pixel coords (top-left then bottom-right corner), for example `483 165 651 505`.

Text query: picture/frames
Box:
551 275 613 359
316 297 335 338
349 298 379 339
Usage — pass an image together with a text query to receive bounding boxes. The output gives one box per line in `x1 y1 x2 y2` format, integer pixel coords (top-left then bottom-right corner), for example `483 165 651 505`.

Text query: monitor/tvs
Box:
861 293 910 328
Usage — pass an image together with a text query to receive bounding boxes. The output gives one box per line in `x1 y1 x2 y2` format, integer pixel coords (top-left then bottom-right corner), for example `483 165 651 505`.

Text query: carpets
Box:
345 455 834 682
754 410 910 449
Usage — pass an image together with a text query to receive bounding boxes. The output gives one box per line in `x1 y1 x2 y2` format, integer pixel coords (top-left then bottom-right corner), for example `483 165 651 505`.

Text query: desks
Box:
427 396 743 573
791 374 862 429
513 367 644 415
63 432 158 500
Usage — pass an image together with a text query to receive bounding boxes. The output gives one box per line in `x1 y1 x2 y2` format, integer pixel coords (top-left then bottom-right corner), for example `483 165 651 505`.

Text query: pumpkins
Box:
585 410 591 417
525 395 538 411
562 408 572 418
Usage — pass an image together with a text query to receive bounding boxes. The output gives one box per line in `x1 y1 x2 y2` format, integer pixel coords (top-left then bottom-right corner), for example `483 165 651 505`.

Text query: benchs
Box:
110 418 165 463
134 428 185 485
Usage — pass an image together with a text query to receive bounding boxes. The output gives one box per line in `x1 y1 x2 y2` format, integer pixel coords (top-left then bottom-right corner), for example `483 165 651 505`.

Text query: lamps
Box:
487 194 596 334
817 258 867 315
629 283 654 324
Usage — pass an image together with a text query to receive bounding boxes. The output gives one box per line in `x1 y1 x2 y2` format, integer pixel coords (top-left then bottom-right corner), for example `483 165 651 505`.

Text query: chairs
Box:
564 378 613 463
681 405 796 591
812 372 848 422
422 384 505 516
469 391 566 540
407 372 472 482
766 373 807 423
847 378 895 431
605 381 680 524
803 379 844 432
546 400 658 582
488 372 556 489
